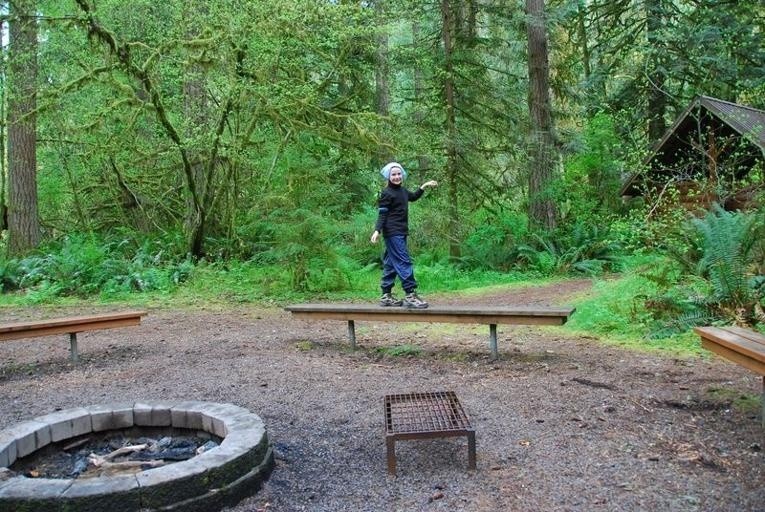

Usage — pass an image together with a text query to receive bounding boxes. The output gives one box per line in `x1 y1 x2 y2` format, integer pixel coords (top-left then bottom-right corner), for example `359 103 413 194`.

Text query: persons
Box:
371 161 438 308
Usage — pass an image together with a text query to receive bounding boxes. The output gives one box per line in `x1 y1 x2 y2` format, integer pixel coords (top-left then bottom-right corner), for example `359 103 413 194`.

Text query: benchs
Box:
695 325 765 431
284 303 577 356
0 311 148 363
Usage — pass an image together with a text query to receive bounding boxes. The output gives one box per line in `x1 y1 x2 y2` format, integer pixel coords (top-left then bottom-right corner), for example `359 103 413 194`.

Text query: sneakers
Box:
380 293 403 306
403 292 428 309
